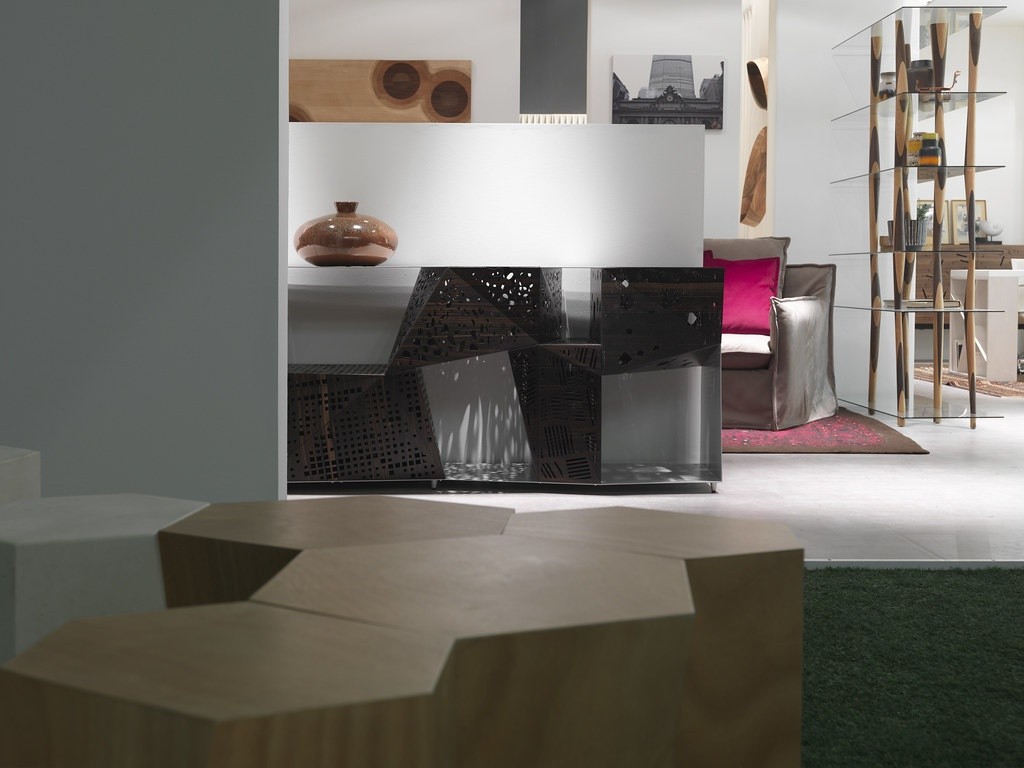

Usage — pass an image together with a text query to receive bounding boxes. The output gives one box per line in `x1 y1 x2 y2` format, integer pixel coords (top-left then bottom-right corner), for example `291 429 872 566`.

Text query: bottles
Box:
918 133 942 166
878 71 897 99
906 131 925 167
292 201 397 266
907 60 934 92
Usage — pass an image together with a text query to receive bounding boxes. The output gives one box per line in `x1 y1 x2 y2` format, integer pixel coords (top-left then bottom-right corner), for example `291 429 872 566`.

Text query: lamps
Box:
745 56 768 111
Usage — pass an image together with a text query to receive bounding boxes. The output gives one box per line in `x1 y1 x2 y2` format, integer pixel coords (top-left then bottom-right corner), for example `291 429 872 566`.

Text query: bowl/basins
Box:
886 219 927 251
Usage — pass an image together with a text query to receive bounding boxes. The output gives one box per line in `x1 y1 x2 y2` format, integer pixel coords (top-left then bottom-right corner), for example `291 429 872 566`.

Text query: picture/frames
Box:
916 200 951 245
951 201 988 245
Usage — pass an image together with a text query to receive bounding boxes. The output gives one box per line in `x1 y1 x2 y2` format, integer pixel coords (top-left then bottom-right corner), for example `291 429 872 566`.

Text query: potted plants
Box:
888 203 931 251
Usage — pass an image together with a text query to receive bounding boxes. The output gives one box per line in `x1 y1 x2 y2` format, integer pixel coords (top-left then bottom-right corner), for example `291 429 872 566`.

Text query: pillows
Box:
703 250 781 336
702 235 792 297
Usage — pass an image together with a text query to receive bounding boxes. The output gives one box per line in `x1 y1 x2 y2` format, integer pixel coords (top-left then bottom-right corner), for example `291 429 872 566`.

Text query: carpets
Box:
913 362 1024 397
799 566 1024 768
722 403 931 455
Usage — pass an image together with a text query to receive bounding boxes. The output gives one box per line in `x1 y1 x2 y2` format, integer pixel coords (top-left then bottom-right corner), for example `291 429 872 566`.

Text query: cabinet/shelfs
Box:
915 244 1024 330
283 265 726 494
830 3 1008 430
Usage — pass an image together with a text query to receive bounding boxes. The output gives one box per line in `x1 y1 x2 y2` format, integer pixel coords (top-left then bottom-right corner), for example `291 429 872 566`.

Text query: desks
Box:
949 269 1024 381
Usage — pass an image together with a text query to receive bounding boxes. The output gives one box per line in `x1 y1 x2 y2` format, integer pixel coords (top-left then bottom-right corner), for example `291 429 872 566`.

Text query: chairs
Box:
720 263 839 431
